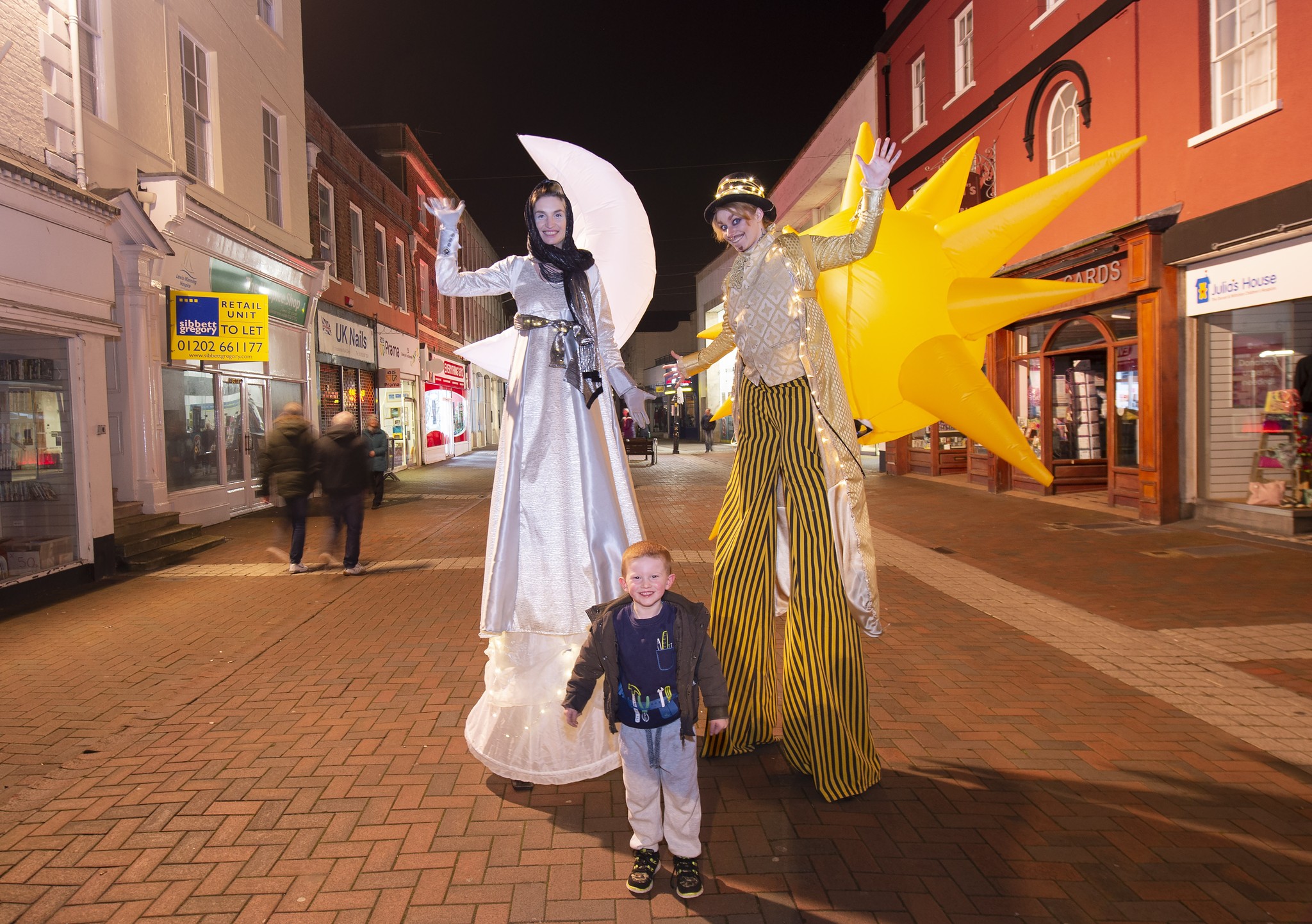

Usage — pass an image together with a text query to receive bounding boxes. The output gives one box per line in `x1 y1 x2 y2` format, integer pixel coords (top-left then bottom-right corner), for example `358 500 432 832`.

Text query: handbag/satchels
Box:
1264 388 1302 411
1246 480 1308 507
648 432 651 438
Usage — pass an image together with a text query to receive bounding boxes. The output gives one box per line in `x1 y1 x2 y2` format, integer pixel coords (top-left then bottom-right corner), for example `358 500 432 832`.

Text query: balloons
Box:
696 120 1148 488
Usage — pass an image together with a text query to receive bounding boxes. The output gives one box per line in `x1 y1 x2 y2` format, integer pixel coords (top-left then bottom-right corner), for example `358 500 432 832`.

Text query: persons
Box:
661 138 903 803
201 424 215 473
1294 353 1312 437
310 411 374 576
617 412 650 452
423 180 658 790
561 540 730 898
360 414 388 509
701 409 716 453
257 401 317 573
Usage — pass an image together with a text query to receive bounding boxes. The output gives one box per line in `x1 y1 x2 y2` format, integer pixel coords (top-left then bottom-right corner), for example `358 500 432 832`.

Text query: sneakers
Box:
343 562 366 575
289 560 308 572
625 848 661 894
320 554 337 564
670 855 704 899
266 547 288 561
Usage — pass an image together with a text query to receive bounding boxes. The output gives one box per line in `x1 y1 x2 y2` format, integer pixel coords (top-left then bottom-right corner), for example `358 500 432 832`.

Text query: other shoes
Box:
512 779 535 790
705 450 709 453
371 504 379 509
711 449 714 452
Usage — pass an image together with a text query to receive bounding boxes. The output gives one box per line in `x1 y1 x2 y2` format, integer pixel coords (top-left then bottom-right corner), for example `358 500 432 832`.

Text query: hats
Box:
368 414 379 422
703 172 777 223
334 411 357 427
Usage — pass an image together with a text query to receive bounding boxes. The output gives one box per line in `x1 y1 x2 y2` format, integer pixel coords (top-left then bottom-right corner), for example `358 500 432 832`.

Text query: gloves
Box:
623 388 657 429
423 196 466 230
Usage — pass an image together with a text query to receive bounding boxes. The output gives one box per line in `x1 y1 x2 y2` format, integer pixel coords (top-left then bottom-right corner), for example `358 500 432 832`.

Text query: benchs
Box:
623 437 657 466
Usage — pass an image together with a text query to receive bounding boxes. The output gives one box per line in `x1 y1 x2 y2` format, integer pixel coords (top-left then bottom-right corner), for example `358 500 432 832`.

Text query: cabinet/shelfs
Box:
1249 410 1301 504
